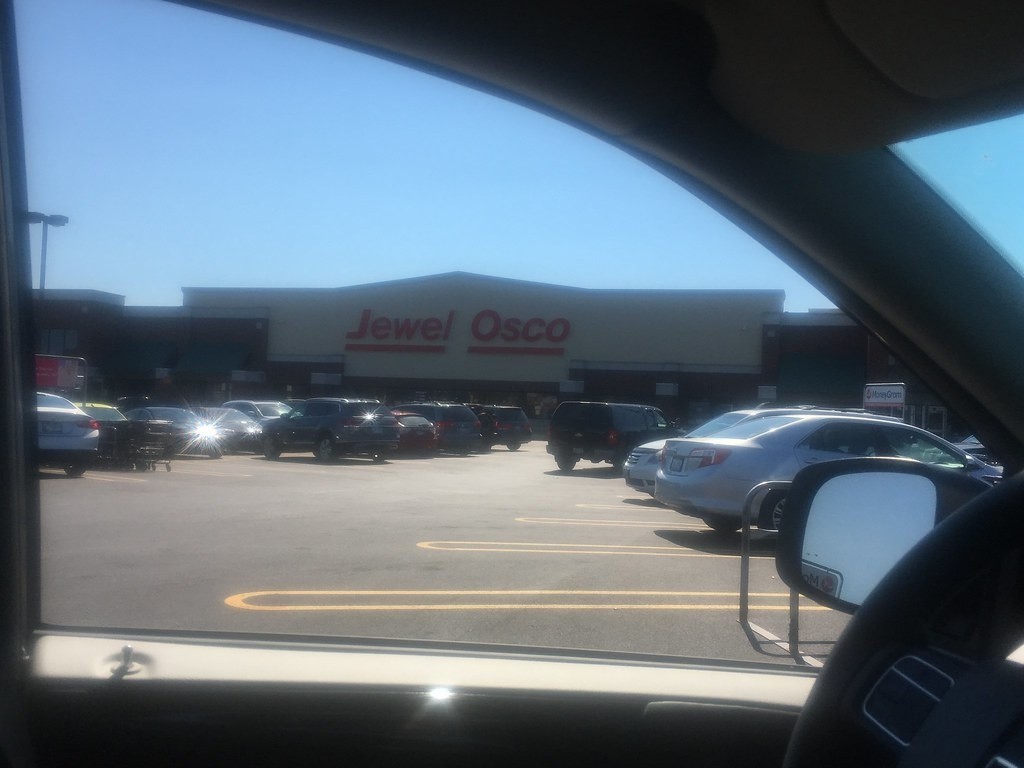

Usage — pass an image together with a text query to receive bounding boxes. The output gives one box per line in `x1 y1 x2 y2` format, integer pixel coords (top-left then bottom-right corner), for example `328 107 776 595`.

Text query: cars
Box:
622 398 917 498
36 392 101 477
70 403 128 421
185 403 264 455
655 414 1004 550
390 409 438 454
922 432 995 466
122 407 228 458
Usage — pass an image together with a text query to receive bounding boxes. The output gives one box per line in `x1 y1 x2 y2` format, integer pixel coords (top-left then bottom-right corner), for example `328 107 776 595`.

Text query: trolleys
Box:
99 419 173 472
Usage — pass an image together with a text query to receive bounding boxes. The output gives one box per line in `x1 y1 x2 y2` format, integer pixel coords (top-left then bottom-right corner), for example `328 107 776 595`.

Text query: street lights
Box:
28 210 69 352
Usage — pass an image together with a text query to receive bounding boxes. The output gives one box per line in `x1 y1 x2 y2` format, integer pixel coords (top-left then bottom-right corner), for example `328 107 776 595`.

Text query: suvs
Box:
389 400 482 456
546 401 688 475
221 398 303 426
259 398 400 464
464 403 534 452
